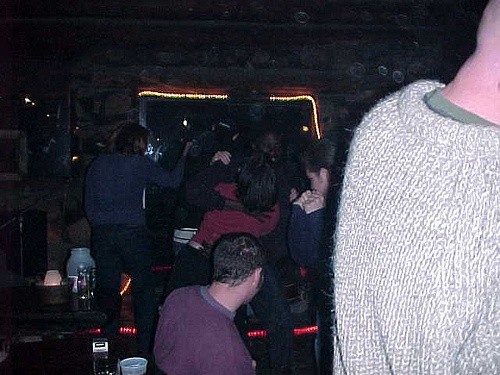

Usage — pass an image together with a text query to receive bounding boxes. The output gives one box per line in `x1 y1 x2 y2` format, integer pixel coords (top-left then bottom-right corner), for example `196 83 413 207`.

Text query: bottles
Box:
78 268 96 312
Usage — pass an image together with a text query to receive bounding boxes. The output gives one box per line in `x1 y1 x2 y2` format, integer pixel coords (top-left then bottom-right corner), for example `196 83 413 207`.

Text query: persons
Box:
80 120 343 375
332 1 500 375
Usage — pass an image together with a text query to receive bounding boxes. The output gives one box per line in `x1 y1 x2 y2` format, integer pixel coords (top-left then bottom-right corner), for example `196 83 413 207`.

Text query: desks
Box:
0 285 122 346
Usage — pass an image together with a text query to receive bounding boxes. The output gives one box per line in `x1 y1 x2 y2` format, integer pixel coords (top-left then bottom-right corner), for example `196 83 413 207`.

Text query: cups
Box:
119 357 148 375
